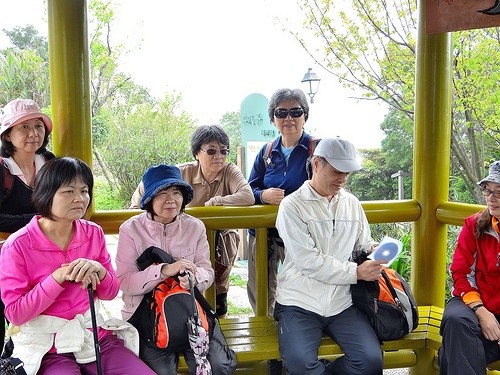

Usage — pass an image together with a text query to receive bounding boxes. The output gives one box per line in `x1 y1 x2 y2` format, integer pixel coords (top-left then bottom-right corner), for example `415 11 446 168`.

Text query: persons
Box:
436 160 500 375
114 163 238 375
1 155 159 375
127 124 257 321
247 89 322 374
0 97 57 359
274 134 388 375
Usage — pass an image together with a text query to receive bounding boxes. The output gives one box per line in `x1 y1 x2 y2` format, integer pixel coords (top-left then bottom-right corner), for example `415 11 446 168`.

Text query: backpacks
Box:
133 247 217 354
350 251 420 343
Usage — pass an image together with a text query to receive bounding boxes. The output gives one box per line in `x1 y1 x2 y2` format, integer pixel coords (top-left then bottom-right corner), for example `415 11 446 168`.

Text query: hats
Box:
312 135 367 174
138 163 195 210
0 99 53 136
476 161 500 185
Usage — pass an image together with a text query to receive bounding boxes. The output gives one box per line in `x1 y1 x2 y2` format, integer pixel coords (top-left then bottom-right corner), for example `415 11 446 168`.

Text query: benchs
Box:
176 305 499 374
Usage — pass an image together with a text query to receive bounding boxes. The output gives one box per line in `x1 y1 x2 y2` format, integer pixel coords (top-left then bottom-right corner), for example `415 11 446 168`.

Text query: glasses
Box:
273 107 305 119
479 187 500 199
200 147 231 156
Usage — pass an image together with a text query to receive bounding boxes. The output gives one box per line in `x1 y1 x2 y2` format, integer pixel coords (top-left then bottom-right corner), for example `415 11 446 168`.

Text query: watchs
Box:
471 303 484 313
